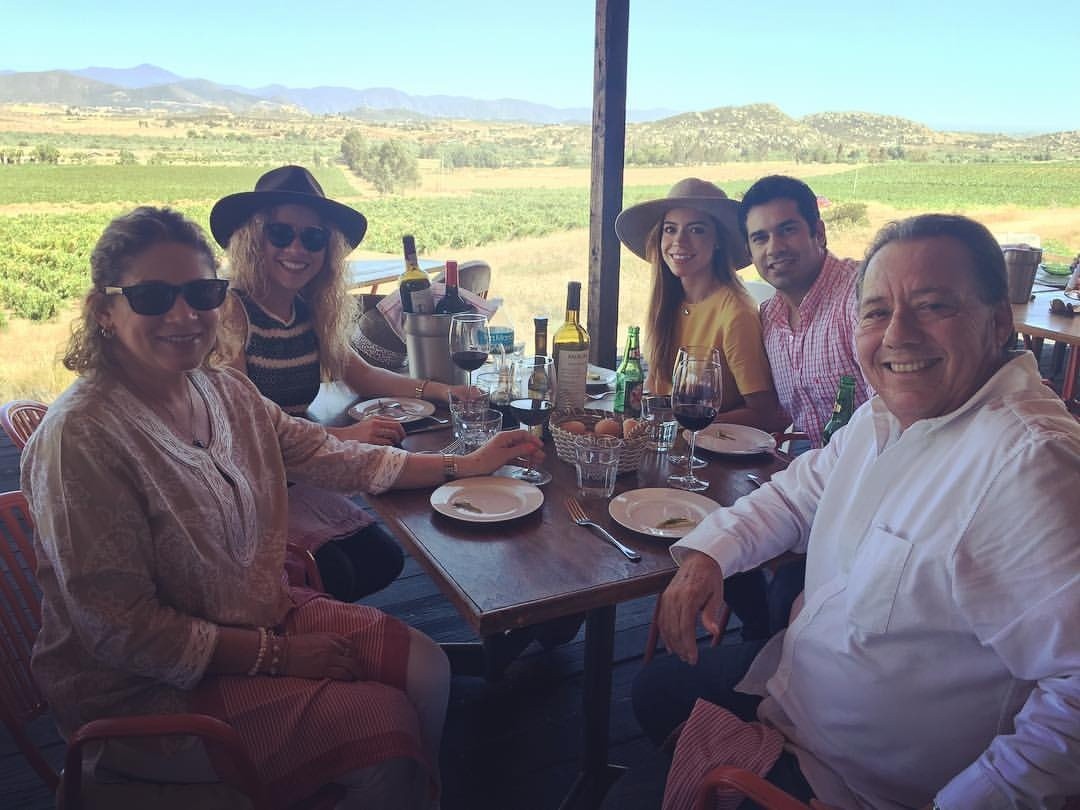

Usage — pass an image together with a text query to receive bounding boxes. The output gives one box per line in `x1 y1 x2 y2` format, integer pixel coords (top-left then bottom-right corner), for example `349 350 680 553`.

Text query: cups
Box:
573 435 621 498
476 373 521 431
452 407 503 456
447 386 490 440
640 396 678 452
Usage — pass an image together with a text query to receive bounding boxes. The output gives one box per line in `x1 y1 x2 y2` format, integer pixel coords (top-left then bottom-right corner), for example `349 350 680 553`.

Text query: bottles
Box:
613 325 643 418
436 260 467 314
552 280 591 408
399 234 434 313
477 295 515 375
528 317 548 442
823 376 857 447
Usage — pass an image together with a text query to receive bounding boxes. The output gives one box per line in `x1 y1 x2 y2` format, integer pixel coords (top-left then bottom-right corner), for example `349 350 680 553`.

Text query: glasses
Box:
100 278 229 317
264 222 328 251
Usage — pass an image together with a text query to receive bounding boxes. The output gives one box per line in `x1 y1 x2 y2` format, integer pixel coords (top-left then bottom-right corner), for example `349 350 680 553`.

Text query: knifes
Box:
406 424 452 434
744 472 767 486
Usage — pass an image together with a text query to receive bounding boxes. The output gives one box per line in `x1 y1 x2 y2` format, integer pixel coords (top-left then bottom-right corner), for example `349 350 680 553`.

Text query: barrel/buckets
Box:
999 244 1042 304
403 307 470 386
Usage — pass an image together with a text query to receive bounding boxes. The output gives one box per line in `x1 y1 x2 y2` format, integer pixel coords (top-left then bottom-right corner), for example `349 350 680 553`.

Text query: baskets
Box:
548 391 650 474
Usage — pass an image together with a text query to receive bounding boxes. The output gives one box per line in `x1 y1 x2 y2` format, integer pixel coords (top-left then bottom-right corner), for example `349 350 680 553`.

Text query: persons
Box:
17 206 549 810
656 212 1080 810
722 177 878 643
468 178 788 682
209 164 489 601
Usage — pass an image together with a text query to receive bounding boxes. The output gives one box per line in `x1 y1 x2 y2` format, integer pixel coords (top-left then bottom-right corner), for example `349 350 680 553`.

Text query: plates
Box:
608 488 723 538
412 414 416 415
682 424 777 456
348 397 435 424
586 374 616 385
430 476 544 522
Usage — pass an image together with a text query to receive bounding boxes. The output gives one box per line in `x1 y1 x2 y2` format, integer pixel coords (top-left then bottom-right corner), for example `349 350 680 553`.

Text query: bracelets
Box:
246 625 268 676
444 453 456 481
415 378 431 400
266 628 282 678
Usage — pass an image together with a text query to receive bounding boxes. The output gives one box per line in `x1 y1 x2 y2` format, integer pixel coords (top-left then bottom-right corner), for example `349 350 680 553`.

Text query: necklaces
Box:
680 303 689 315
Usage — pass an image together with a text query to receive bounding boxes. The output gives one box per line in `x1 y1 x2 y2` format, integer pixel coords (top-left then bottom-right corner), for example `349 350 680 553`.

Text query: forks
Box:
586 391 616 399
417 439 462 454
566 495 641 560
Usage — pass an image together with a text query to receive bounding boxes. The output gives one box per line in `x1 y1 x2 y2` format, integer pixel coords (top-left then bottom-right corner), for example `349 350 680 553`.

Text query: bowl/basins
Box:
1040 264 1073 275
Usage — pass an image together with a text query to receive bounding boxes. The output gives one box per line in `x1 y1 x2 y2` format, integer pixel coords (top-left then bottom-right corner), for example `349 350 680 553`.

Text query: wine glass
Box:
666 344 721 491
507 355 560 485
448 314 491 385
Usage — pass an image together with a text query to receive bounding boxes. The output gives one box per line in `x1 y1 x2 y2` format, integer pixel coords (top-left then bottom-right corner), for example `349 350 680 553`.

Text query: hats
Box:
209 165 368 258
615 177 753 271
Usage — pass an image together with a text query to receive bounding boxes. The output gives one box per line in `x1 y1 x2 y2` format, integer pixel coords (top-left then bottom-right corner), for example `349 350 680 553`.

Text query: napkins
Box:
184 597 439 809
285 484 375 585
662 699 786 810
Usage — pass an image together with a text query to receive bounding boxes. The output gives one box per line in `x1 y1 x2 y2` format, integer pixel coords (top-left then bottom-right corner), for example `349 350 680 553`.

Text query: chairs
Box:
0 398 50 449
431 260 492 299
0 490 324 810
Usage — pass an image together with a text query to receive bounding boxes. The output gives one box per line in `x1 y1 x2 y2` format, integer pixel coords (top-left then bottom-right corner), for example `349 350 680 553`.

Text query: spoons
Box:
383 401 449 424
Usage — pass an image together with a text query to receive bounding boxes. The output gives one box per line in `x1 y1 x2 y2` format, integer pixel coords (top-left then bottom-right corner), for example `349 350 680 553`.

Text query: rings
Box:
344 647 351 656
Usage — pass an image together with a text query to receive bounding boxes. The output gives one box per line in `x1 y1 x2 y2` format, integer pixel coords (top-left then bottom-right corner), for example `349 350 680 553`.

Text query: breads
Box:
557 418 639 439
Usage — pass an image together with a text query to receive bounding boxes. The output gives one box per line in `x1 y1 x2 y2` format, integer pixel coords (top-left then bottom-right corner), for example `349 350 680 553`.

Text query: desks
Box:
305 350 798 810
1008 270 1080 404
344 257 447 296
